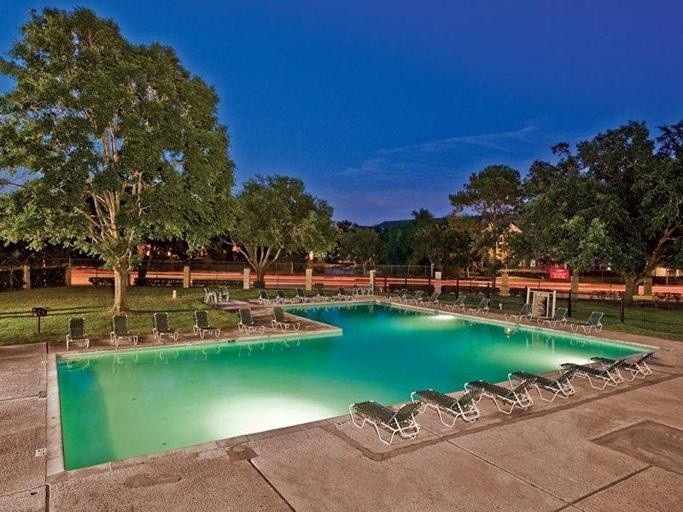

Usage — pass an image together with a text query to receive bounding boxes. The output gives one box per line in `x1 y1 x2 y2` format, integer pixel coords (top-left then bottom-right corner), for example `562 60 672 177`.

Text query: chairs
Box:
389 285 604 333
66 306 301 352
203 286 230 304
257 284 354 306
